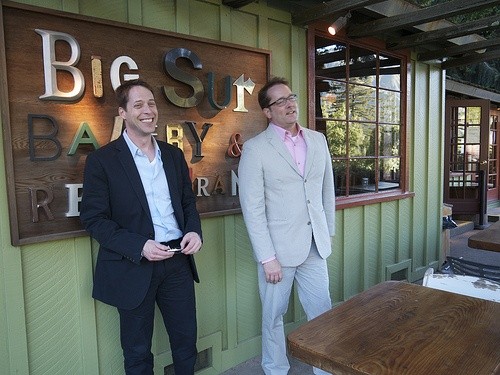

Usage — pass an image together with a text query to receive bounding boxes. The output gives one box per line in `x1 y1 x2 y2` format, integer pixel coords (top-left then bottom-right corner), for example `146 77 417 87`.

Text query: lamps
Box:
328 16 347 35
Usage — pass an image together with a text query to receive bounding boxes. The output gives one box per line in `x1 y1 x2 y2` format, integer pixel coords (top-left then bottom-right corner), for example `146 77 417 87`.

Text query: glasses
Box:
267 93 296 108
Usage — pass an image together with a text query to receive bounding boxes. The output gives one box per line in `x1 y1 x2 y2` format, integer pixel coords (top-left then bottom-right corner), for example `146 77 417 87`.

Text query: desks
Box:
468 220 500 252
287 280 500 375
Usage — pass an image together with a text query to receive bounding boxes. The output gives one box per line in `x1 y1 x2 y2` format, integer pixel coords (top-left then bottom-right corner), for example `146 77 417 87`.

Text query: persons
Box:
237 77 336 375
79 78 204 375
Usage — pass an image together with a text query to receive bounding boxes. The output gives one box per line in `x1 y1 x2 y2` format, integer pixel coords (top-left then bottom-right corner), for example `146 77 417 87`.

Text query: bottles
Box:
361 174 368 189
